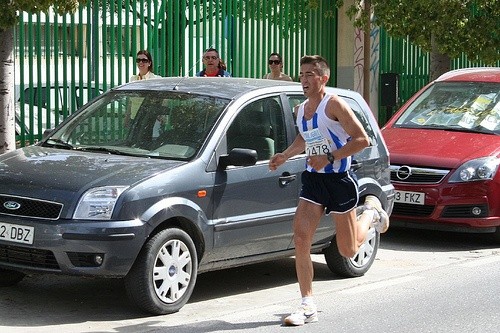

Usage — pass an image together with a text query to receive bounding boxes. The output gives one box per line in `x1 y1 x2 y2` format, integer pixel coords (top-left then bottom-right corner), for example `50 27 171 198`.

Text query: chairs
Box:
229 111 276 157
153 103 201 147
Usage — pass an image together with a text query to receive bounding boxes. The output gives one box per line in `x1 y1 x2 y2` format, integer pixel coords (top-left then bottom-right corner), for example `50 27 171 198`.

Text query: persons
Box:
262 53 293 82
196 48 231 77
125 50 167 139
268 55 389 325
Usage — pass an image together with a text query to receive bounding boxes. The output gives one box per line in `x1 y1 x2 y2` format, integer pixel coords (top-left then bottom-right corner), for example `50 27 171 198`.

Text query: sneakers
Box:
285 303 318 326
365 196 389 232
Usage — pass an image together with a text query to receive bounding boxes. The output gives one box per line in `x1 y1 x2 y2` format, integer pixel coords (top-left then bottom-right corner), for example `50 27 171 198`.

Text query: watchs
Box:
327 152 335 165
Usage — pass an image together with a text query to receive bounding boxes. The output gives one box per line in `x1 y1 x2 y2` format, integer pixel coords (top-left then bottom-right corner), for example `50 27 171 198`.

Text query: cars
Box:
380 67 500 240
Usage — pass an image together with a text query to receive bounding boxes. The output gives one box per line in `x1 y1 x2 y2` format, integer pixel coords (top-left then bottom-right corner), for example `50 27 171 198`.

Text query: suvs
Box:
14 83 115 138
1 45 394 315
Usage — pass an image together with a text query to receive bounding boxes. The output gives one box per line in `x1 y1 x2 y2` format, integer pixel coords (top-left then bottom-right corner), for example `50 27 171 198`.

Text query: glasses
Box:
136 58 148 63
268 60 281 65
